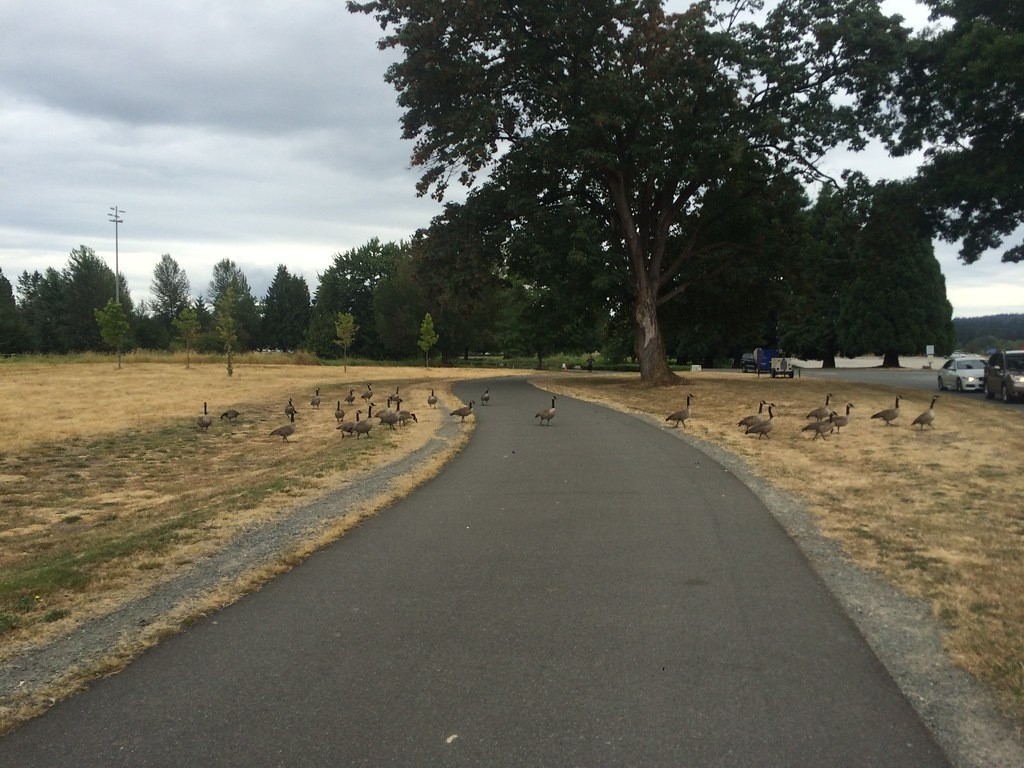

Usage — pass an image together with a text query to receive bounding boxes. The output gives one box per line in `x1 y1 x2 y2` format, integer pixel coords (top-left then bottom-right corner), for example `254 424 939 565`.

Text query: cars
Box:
938 357 988 393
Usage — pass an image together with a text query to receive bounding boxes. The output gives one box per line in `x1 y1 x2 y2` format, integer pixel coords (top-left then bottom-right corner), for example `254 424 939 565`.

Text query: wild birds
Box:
481 385 490 406
801 411 837 441
220 411 240 422
737 401 768 432
806 394 832 421
427 389 437 409
197 402 212 432
337 411 361 438
745 403 776 440
666 394 697 428
826 403 856 434
450 400 475 422
378 408 419 430
353 403 377 440
871 395 904 427
912 395 941 429
270 409 299 443
283 383 373 422
535 396 557 425
388 385 401 408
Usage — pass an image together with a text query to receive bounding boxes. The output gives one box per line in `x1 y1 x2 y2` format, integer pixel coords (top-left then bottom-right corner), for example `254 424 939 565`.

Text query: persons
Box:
562 362 568 370
586 356 596 372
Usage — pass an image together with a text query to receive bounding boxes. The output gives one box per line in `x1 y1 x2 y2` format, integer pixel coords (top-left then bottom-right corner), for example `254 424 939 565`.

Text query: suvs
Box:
740 353 757 373
982 348 1024 403
